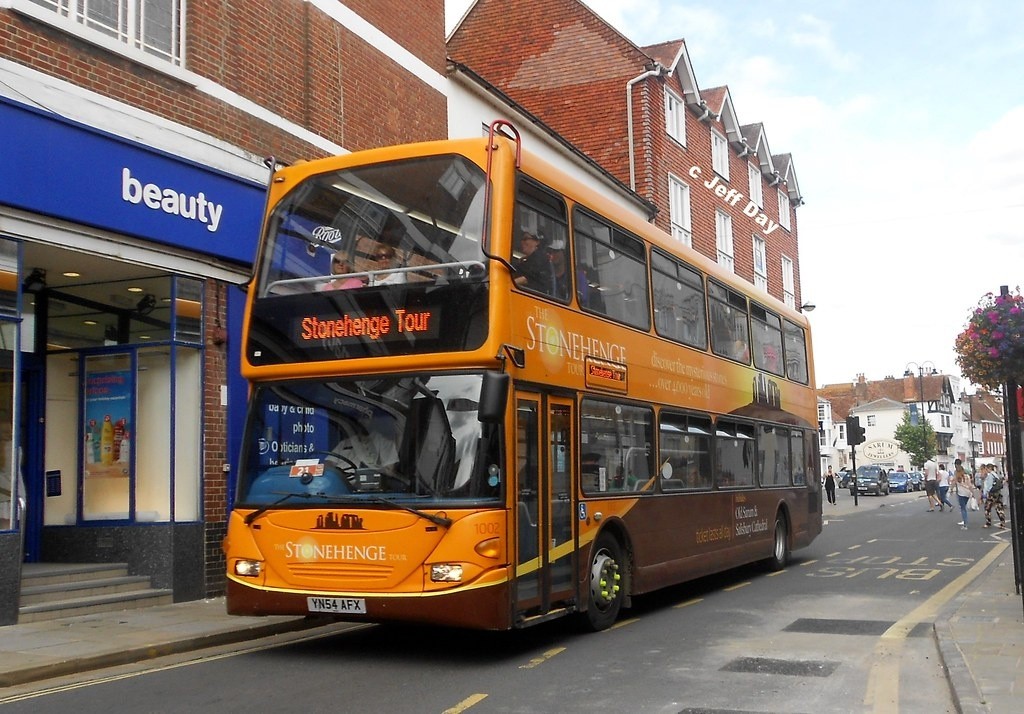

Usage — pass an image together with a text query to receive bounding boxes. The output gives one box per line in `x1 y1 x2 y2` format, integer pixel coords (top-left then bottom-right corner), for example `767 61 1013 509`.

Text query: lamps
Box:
137 294 156 315
24 268 47 291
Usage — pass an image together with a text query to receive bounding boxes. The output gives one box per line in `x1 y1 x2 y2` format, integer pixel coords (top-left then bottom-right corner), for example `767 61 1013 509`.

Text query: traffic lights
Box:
853 417 865 445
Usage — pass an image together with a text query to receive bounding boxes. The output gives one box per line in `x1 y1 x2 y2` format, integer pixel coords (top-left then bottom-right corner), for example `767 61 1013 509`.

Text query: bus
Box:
223 120 822 631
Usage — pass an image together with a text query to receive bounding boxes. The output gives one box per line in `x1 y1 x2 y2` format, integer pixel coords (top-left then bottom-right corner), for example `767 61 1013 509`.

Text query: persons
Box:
975 463 1010 529
321 250 363 291
924 455 955 512
548 240 588 306
515 229 552 297
323 404 399 485
763 333 777 370
581 453 815 490
950 459 975 530
366 243 406 286
822 465 836 505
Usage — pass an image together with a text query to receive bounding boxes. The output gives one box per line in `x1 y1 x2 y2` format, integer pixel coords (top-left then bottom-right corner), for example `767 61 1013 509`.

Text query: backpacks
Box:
990 473 1003 492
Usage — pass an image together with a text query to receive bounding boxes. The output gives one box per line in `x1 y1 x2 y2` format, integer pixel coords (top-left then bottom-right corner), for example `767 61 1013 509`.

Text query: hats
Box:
548 239 566 250
520 226 546 243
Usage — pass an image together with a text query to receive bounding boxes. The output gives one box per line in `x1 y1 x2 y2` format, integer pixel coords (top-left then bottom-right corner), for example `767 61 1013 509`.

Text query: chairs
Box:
545 261 626 319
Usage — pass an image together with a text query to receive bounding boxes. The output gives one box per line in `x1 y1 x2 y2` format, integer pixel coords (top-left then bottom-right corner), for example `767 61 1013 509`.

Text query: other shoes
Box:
961 526 969 531
983 524 992 528
957 520 966 524
998 525 1006 529
939 505 946 511
950 505 955 512
926 508 934 512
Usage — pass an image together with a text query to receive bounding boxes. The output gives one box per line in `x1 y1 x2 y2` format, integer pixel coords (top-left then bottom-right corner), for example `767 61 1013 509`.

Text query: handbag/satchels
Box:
967 495 980 511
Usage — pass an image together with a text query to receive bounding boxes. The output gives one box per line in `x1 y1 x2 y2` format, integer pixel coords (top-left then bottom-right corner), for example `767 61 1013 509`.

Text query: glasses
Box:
375 254 392 261
521 237 535 241
334 258 349 264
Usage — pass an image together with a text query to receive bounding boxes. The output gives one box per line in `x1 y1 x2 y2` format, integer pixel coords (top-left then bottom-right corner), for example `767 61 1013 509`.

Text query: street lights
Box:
904 361 939 461
958 391 984 485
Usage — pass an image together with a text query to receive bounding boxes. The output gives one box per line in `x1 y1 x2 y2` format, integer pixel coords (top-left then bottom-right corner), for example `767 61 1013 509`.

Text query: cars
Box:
835 465 982 496
